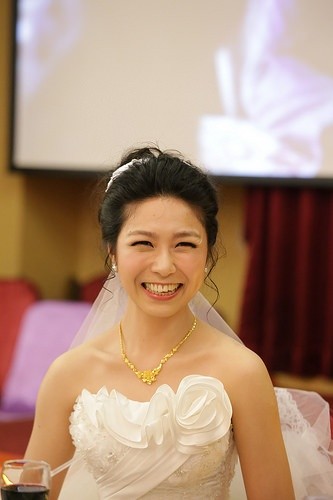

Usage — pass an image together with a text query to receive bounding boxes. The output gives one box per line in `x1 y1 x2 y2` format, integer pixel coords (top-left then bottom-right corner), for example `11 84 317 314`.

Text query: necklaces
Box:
117 316 198 386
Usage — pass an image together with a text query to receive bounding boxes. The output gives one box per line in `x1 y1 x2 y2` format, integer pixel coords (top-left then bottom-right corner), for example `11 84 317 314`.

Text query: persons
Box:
18 147 297 500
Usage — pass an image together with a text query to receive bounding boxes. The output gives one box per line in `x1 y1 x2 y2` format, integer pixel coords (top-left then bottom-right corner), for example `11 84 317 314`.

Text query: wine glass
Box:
0 459 51 500
